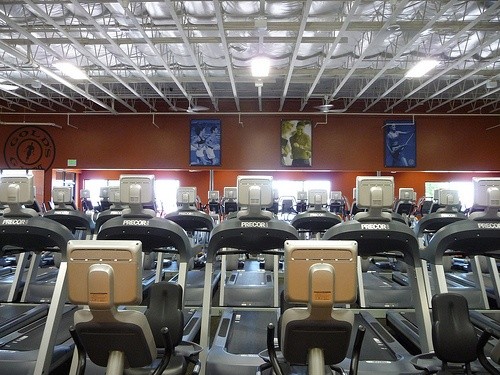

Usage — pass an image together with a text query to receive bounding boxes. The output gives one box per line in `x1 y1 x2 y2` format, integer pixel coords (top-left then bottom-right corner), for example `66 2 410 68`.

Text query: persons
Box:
192 124 218 165
281 121 312 167
386 125 412 167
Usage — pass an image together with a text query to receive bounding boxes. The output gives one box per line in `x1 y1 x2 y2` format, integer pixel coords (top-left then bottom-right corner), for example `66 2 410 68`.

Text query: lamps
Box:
404 54 442 78
51 59 89 80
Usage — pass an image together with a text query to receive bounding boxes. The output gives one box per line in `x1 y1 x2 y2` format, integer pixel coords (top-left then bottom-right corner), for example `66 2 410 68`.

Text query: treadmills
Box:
0 175 499 375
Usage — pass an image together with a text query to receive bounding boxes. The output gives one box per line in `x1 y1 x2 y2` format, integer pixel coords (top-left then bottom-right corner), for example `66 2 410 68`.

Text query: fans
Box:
169 95 210 113
313 96 346 113
